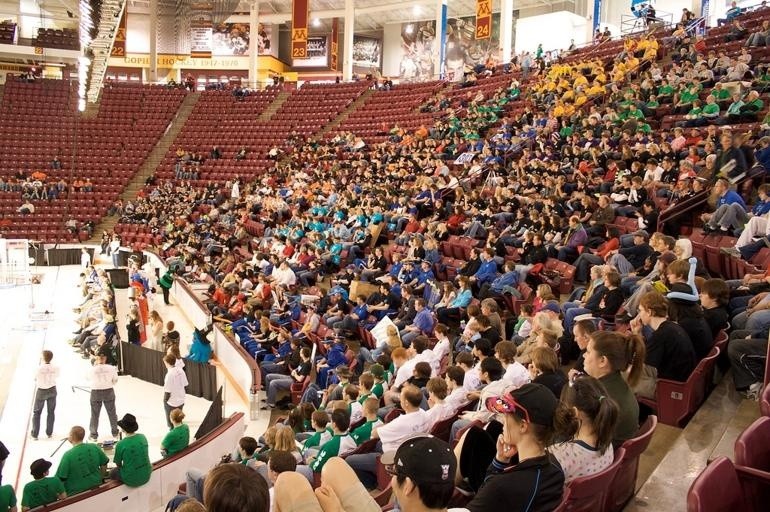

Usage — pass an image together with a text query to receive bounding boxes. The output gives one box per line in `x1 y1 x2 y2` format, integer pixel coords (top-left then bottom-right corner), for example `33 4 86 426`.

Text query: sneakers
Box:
746 382 763 402
261 404 276 410
261 398 269 402
720 246 741 259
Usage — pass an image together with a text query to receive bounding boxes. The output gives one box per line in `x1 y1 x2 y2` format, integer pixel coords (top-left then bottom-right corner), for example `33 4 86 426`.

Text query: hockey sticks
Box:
71 385 92 393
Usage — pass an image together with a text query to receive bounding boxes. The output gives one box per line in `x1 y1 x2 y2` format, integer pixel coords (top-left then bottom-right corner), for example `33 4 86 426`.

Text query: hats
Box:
633 229 649 239
486 384 557 424
380 434 457 484
117 413 137 431
30 460 51 475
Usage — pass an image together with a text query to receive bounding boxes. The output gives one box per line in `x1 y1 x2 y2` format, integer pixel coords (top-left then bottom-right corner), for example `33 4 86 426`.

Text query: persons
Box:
1 0 770 512
